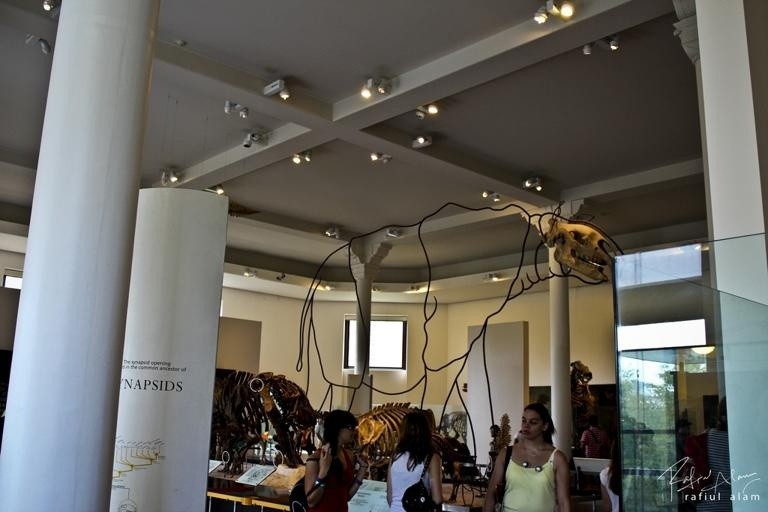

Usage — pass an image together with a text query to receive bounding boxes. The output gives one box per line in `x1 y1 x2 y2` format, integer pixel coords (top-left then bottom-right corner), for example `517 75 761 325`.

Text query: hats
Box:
323 410 359 428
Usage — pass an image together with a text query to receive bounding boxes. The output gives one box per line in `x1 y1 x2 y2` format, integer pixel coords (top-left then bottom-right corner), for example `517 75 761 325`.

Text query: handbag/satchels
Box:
599 444 611 458
401 450 440 512
494 445 514 504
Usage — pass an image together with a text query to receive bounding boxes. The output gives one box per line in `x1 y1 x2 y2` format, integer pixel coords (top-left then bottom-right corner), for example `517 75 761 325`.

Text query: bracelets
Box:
360 465 368 468
356 477 363 486
315 478 326 487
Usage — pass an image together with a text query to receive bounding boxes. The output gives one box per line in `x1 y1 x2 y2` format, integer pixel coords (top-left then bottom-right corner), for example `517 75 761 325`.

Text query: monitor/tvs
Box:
344 319 407 369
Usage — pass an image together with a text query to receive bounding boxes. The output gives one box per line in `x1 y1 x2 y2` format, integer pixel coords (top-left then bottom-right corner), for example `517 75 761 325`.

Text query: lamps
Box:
522 175 544 193
325 227 340 240
362 78 440 163
533 0 574 25
221 79 291 149
482 190 500 203
161 169 179 186
292 148 313 165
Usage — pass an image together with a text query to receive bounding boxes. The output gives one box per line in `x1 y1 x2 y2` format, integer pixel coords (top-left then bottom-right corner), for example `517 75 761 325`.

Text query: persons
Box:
387 412 443 512
695 396 733 512
580 414 620 512
483 401 570 512
304 409 367 511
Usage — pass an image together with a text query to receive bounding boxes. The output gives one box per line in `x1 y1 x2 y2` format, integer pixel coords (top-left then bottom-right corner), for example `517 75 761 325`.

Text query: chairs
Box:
450 454 489 506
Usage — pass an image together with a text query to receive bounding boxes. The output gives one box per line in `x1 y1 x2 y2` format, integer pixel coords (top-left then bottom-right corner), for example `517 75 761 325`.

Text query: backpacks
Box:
289 458 327 512
